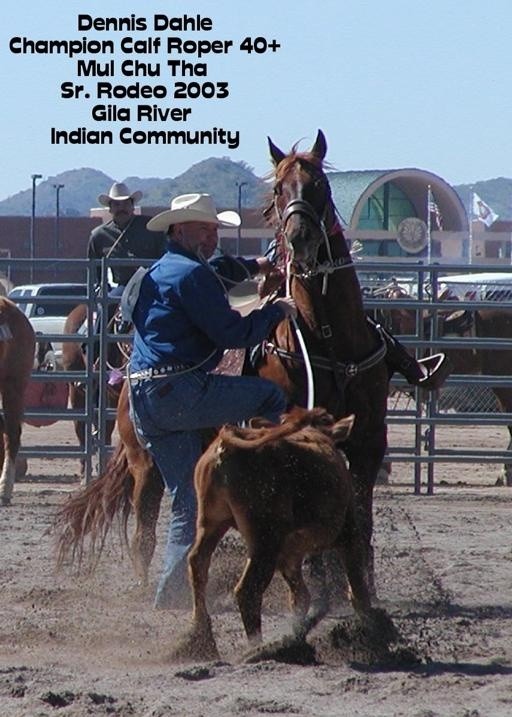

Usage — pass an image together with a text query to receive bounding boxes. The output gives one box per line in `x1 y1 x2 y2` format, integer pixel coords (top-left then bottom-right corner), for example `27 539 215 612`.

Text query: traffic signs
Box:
52 183 66 259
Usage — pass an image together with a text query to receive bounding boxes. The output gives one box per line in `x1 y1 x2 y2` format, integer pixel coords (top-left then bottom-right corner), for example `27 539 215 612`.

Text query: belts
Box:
128 362 194 380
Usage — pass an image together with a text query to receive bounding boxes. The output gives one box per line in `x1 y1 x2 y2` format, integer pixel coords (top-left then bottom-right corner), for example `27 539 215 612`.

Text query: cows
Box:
186 403 375 663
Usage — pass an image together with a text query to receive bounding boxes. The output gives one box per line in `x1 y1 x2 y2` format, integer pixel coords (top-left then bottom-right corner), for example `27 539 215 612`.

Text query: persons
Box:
116 192 299 616
84 180 168 385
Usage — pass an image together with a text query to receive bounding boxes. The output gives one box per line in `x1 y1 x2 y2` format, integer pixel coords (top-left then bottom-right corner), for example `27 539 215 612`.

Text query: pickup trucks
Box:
7 282 102 361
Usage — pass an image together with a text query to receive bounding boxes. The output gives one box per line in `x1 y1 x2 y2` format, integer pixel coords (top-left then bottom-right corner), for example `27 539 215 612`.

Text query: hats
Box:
98 181 144 206
145 193 242 232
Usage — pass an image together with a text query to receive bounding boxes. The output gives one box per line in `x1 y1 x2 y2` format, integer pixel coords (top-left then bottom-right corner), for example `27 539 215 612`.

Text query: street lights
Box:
29 172 43 284
234 179 248 265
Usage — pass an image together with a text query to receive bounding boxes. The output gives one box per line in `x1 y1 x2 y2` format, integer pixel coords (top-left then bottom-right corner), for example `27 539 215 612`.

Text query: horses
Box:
0 294 37 512
62 300 127 488
392 279 512 487
38 126 394 620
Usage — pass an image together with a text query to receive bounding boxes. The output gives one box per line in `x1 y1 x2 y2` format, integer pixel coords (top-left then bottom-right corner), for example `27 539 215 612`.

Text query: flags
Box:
470 191 500 228
428 189 445 232
